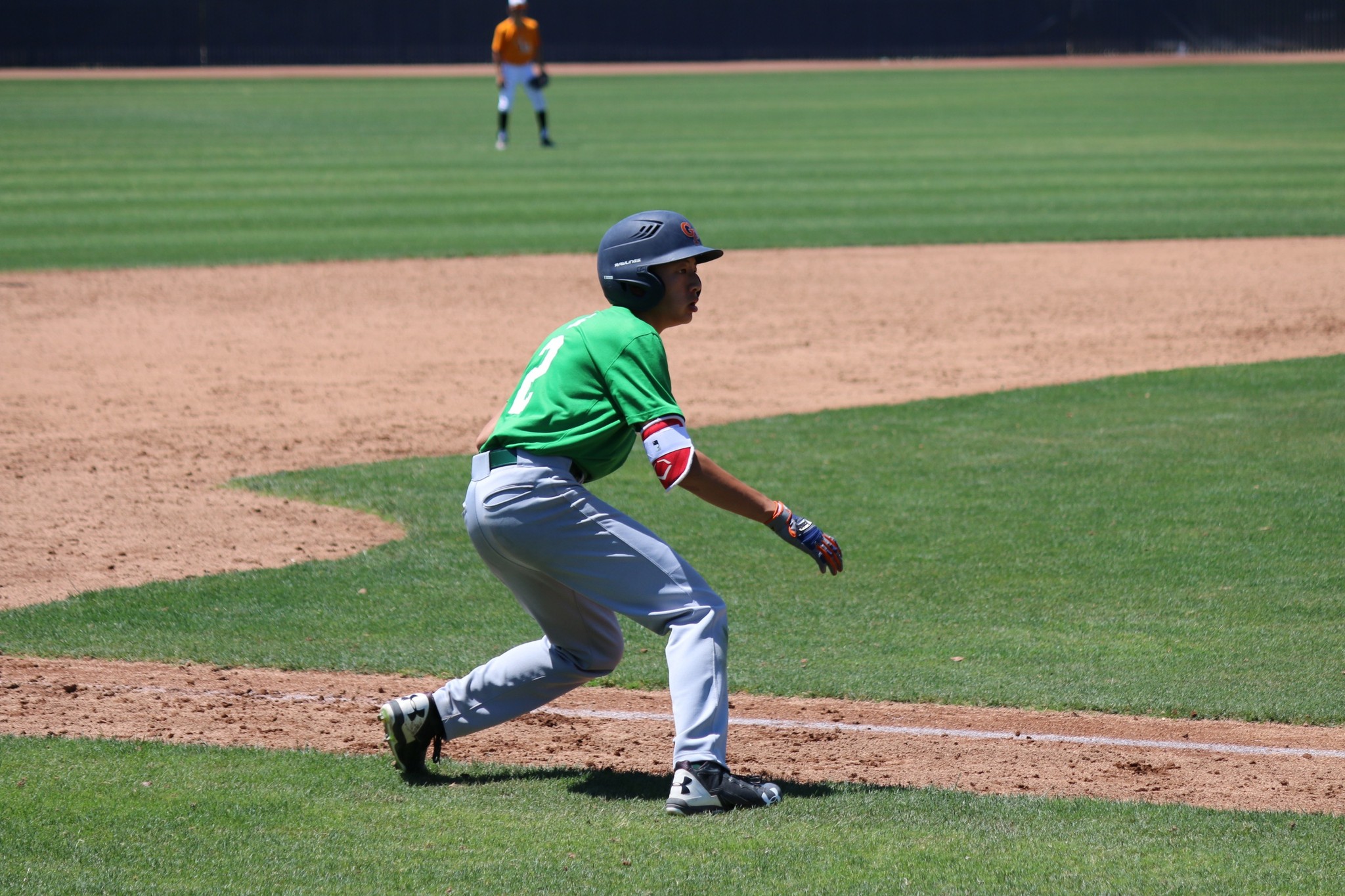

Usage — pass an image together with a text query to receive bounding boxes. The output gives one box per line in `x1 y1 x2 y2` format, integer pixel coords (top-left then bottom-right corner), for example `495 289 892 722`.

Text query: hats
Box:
508 0 528 10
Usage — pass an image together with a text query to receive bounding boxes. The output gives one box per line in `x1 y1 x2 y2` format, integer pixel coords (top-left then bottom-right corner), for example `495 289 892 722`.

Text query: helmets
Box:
597 211 723 311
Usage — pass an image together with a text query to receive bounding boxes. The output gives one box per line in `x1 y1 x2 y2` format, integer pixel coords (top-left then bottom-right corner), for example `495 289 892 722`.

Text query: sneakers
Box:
378 692 437 783
666 761 782 816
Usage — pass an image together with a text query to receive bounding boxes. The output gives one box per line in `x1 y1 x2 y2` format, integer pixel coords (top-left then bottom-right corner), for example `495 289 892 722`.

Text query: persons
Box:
491 0 557 152
378 209 843 819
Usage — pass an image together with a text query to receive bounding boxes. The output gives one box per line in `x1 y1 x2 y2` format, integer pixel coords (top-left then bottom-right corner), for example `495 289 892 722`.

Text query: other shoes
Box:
497 138 507 149
541 138 551 146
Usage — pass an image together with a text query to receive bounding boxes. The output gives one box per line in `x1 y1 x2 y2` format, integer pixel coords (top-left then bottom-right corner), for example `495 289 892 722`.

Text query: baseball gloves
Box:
530 72 549 89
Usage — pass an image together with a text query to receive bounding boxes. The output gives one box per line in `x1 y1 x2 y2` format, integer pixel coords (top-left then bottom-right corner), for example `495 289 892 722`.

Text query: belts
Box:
489 449 583 482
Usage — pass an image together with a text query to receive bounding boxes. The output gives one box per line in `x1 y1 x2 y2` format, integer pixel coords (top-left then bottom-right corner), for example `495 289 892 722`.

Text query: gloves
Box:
762 501 844 576
